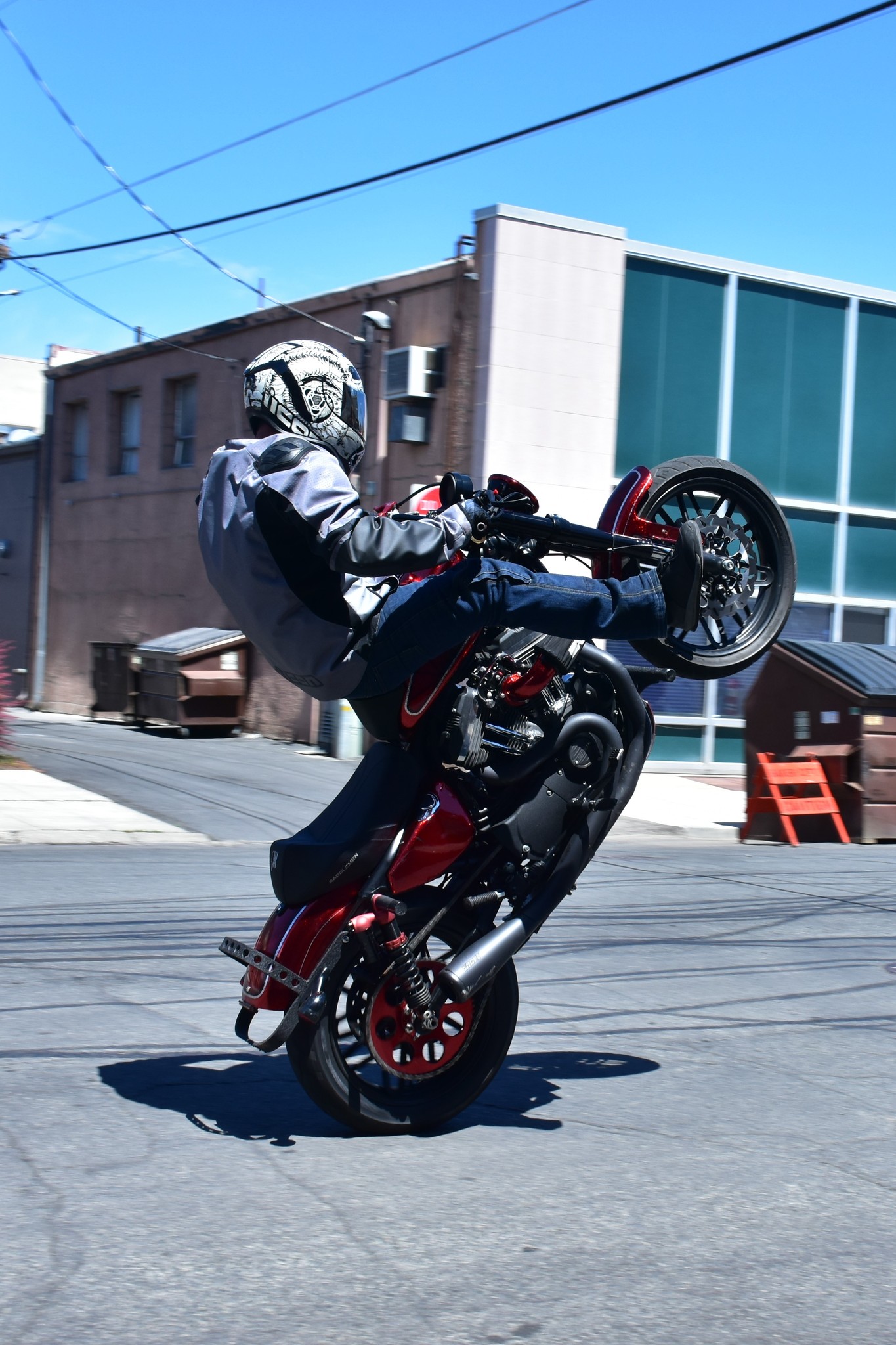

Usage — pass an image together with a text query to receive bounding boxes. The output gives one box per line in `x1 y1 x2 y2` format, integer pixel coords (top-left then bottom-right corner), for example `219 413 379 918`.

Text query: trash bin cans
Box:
126 625 251 732
87 641 135 712
745 639 896 844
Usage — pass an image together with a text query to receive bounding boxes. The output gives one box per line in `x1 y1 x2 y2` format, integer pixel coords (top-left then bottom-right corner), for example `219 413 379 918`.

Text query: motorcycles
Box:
215 455 798 1134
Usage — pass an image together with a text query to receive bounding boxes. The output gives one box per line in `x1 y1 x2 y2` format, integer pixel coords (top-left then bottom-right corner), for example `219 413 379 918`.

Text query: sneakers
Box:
655 520 704 632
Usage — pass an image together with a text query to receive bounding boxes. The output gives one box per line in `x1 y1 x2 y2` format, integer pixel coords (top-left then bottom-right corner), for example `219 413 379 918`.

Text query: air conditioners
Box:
378 340 442 406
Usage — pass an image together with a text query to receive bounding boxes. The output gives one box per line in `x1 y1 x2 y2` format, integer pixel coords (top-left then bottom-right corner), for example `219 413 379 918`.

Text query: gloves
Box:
460 499 492 532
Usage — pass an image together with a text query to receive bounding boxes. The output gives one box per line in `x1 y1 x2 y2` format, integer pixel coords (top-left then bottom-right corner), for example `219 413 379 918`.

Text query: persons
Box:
198 338 703 701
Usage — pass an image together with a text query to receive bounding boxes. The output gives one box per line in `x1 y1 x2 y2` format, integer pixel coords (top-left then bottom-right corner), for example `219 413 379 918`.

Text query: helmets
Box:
243 340 368 477
